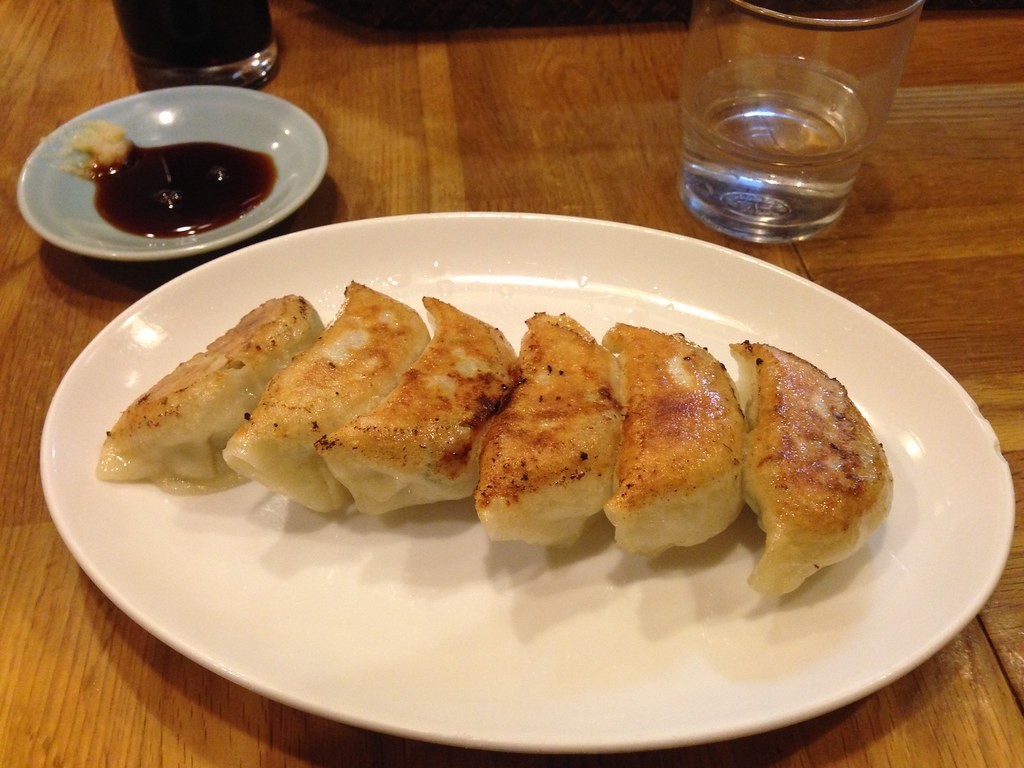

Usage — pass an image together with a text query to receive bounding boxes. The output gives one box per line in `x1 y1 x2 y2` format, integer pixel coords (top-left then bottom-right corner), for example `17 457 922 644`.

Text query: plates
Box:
37 211 1016 756
16 84 330 264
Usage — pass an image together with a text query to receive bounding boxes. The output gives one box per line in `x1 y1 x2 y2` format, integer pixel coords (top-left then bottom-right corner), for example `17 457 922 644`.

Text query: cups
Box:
675 0 922 245
111 0 280 89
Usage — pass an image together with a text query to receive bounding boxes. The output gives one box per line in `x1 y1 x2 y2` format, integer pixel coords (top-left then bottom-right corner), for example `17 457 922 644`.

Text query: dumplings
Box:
96 279 892 599
